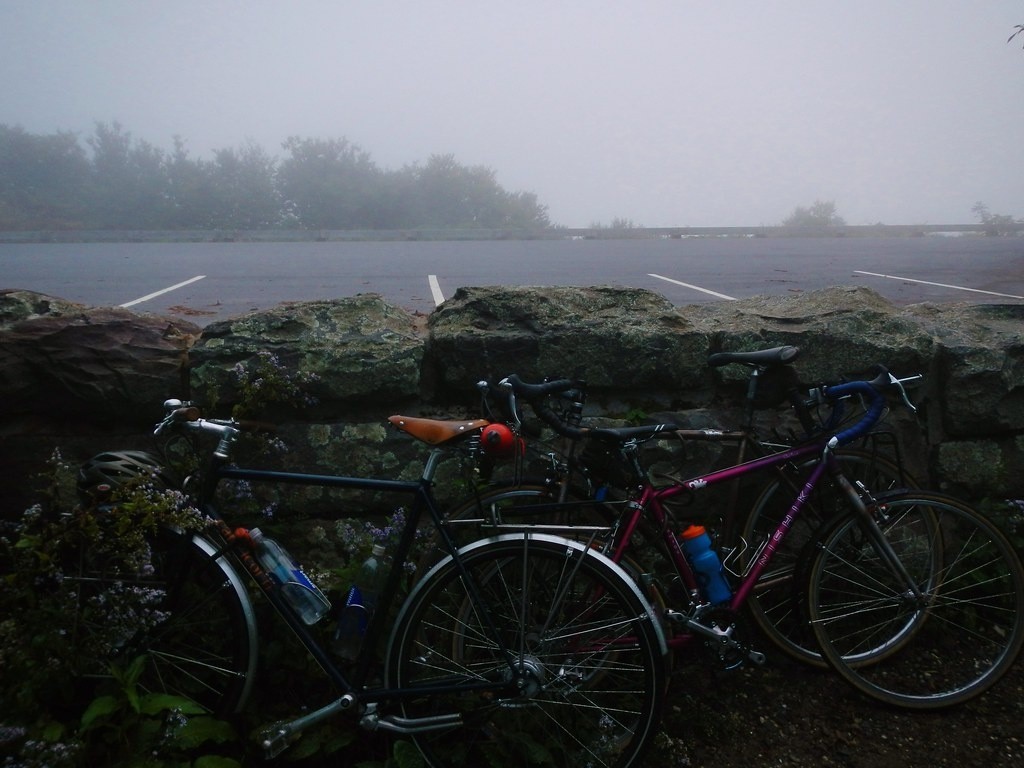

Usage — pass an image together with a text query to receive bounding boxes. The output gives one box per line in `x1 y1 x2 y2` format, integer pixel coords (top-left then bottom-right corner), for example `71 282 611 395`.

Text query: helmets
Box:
77 449 178 509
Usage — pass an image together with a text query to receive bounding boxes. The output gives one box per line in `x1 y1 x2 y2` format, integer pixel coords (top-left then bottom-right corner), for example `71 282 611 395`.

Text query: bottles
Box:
250 527 332 626
481 424 525 463
682 525 733 607
331 544 385 660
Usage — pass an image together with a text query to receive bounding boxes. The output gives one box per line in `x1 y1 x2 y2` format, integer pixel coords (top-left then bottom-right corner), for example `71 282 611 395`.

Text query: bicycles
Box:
17 396 671 768
404 338 1024 729
